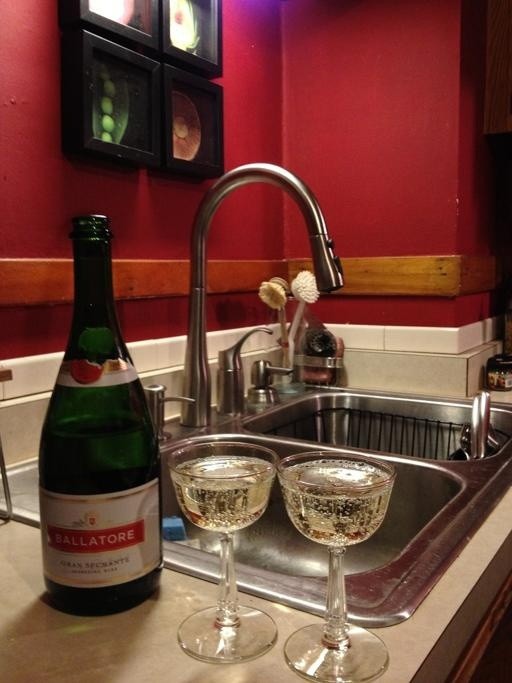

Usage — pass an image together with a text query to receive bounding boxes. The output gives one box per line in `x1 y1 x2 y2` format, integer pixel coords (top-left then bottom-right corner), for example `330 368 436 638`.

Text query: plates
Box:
170 0 199 54
167 92 202 161
93 67 133 145
90 0 137 26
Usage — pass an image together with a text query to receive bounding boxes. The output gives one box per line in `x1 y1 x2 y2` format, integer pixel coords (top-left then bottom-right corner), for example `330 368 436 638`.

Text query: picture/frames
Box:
58 0 228 181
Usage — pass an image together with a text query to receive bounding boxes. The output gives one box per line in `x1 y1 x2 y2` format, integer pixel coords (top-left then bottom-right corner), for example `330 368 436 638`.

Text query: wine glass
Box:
275 449 398 680
167 442 277 666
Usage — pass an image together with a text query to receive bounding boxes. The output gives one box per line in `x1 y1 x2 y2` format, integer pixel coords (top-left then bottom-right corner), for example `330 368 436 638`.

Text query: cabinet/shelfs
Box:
447 571 512 681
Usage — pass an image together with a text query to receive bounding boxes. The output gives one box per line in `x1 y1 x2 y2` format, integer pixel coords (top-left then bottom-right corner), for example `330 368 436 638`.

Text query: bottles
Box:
487 352 511 392
38 217 166 614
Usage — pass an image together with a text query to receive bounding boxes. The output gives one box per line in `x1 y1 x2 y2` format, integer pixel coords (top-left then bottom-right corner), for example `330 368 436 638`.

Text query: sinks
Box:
155 435 476 629
242 391 512 502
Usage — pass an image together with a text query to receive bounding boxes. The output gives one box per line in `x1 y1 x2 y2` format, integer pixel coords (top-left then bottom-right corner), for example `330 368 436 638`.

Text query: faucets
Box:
180 162 342 428
